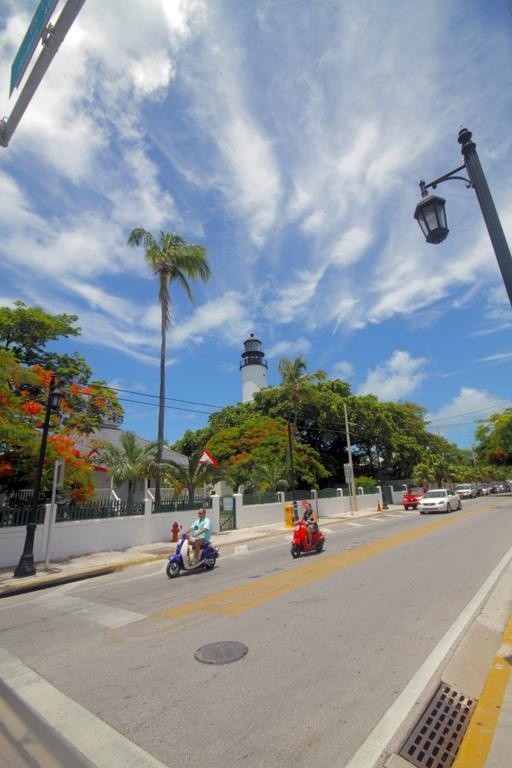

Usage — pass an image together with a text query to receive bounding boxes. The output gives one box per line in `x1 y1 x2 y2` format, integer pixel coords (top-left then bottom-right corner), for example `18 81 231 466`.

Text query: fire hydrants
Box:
170 521 180 543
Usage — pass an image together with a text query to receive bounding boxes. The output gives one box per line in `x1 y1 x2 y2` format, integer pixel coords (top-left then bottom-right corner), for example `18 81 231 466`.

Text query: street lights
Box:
13 374 66 578
345 421 360 513
374 441 389 510
410 125 511 305
286 419 302 524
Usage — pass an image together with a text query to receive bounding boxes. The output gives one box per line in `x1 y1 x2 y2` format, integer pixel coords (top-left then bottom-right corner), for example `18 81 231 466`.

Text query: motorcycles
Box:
289 520 326 558
165 525 220 578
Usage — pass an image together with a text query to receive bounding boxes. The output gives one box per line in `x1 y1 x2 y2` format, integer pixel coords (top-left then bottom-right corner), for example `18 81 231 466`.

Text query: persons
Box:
293 500 318 549
180 506 212 567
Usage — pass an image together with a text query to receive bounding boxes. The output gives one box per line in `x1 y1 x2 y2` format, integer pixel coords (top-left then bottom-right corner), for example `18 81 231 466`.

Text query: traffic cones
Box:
376 499 382 512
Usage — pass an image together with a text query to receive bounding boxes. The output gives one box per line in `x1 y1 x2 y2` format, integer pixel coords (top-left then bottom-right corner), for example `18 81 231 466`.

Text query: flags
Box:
198 447 221 470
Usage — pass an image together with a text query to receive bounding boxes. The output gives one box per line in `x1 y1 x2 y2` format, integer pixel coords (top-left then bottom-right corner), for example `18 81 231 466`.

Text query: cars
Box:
402 483 507 514
